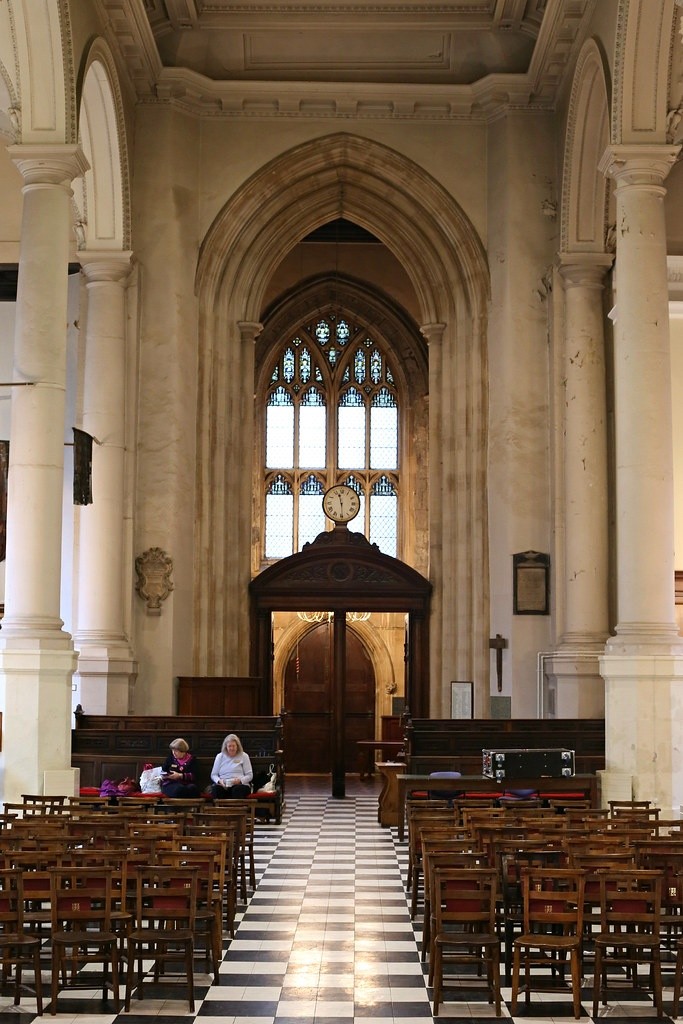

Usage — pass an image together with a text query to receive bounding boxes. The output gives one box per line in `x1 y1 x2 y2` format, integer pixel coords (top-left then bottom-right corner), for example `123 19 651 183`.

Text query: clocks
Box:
322 485 360 522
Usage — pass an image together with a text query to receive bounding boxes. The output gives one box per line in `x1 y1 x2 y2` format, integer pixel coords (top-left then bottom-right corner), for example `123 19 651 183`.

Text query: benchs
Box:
415 788 587 799
76 787 282 823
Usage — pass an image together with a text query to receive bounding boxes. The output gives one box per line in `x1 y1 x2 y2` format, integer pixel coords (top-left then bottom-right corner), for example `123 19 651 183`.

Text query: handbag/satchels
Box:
100 766 162 797
257 764 276 794
161 778 201 799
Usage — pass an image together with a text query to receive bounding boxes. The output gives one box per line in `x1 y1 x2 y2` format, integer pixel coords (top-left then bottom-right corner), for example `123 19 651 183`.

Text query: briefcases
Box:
483 749 575 783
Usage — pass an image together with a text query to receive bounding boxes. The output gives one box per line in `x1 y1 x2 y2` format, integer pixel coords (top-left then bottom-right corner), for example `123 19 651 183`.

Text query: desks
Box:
396 773 601 833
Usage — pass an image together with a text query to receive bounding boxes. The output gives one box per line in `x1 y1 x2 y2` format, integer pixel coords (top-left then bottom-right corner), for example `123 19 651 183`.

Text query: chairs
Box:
404 799 683 1020
0 799 261 1016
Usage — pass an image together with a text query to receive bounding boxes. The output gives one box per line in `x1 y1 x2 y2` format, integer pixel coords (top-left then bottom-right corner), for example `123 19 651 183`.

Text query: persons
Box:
159 738 198 814
205 734 252 799
